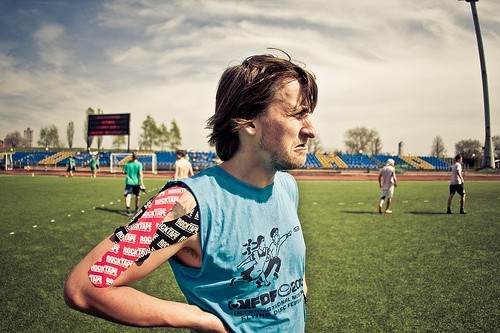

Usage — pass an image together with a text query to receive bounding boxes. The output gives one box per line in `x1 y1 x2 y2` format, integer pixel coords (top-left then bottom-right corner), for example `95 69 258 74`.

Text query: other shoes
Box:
385 211 392 213
127 213 132 216
460 210 467 214
446 211 454 214
378 205 382 214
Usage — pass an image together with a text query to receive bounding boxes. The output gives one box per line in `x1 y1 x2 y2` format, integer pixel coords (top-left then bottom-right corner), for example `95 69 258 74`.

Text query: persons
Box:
63 47 319 333
65 155 76 178
446 154 468 214
83 154 99 178
122 154 145 217
378 159 397 213
173 150 194 180
